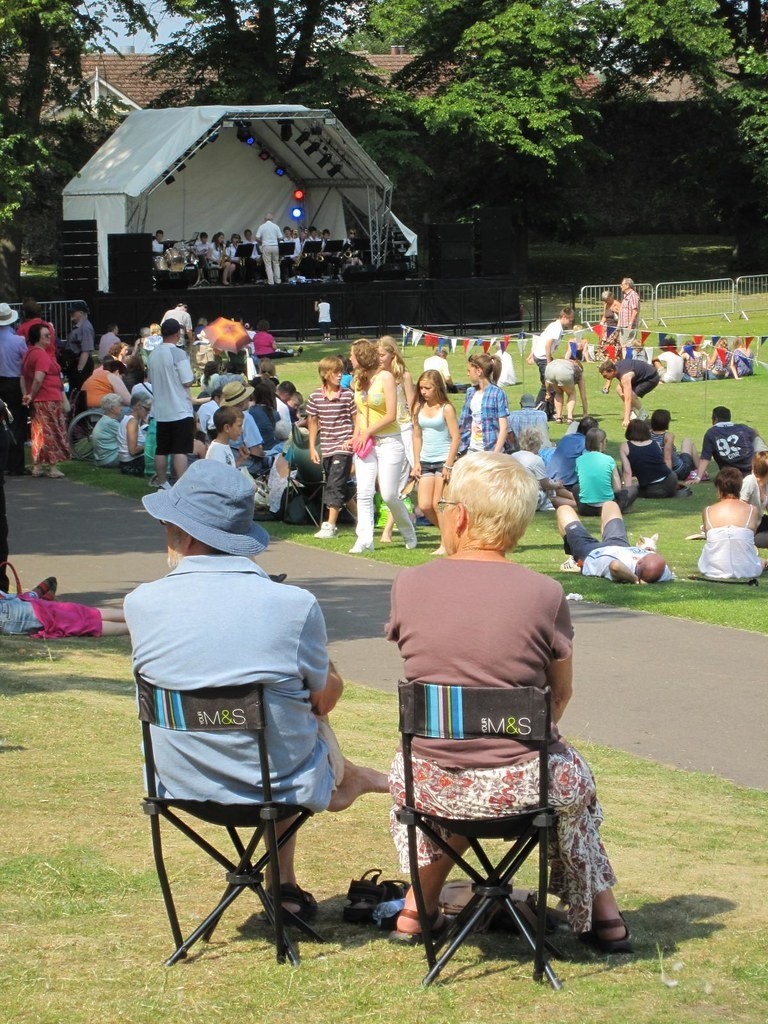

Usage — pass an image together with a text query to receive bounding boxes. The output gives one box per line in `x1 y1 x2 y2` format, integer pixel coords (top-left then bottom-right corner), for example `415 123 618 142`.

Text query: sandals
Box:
581 911 632 952
341 868 410 919
555 418 563 423
266 883 317 921
567 418 575 424
389 906 456 944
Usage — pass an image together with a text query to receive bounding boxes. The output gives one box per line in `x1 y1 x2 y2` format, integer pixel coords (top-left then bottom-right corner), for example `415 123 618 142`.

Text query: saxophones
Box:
295 251 308 268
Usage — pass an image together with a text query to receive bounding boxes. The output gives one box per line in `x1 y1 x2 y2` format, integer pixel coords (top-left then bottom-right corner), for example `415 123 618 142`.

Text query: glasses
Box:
438 497 457 511
143 406 150 411
42 334 51 338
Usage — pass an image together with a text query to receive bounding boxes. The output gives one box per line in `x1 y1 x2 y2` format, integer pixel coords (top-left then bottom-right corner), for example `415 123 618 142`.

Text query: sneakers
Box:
313 522 339 539
560 556 581 572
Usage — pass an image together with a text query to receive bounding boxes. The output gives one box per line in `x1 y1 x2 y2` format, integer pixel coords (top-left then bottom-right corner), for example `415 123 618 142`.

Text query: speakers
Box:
156 271 195 290
60 220 154 295
342 262 407 282
427 206 527 279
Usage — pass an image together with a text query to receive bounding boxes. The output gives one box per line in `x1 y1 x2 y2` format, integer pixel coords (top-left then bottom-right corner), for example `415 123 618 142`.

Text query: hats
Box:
219 381 255 406
66 302 87 313
0 303 19 325
140 458 269 556
161 318 184 333
521 394 536 407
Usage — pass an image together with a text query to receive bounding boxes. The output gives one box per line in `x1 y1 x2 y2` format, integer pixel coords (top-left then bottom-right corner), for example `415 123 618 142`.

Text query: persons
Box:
0 214 768 638
382 452 634 952
122 460 389 925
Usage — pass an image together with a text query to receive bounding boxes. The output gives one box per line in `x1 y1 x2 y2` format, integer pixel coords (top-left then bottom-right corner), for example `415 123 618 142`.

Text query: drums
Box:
153 256 167 269
170 263 200 288
164 247 182 264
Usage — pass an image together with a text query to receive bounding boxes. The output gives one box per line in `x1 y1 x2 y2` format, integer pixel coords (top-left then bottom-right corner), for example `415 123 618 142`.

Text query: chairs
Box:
198 254 220 285
231 447 275 521
281 425 357 529
396 679 568 992
134 671 325 967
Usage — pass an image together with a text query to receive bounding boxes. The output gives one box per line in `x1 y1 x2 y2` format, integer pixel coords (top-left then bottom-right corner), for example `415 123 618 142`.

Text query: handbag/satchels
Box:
353 433 375 458
440 878 535 933
61 392 71 412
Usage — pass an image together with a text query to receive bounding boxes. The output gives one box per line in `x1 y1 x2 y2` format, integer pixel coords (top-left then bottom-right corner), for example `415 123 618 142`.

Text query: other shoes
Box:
50 470 65 478
349 546 374 554
638 411 649 420
404 534 416 549
32 470 46 476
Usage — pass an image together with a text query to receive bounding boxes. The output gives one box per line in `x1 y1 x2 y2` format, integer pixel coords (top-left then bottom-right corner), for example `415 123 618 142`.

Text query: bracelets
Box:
443 464 453 470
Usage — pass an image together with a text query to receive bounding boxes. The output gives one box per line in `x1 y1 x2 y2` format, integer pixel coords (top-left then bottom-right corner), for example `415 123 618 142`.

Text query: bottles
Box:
372 898 406 927
